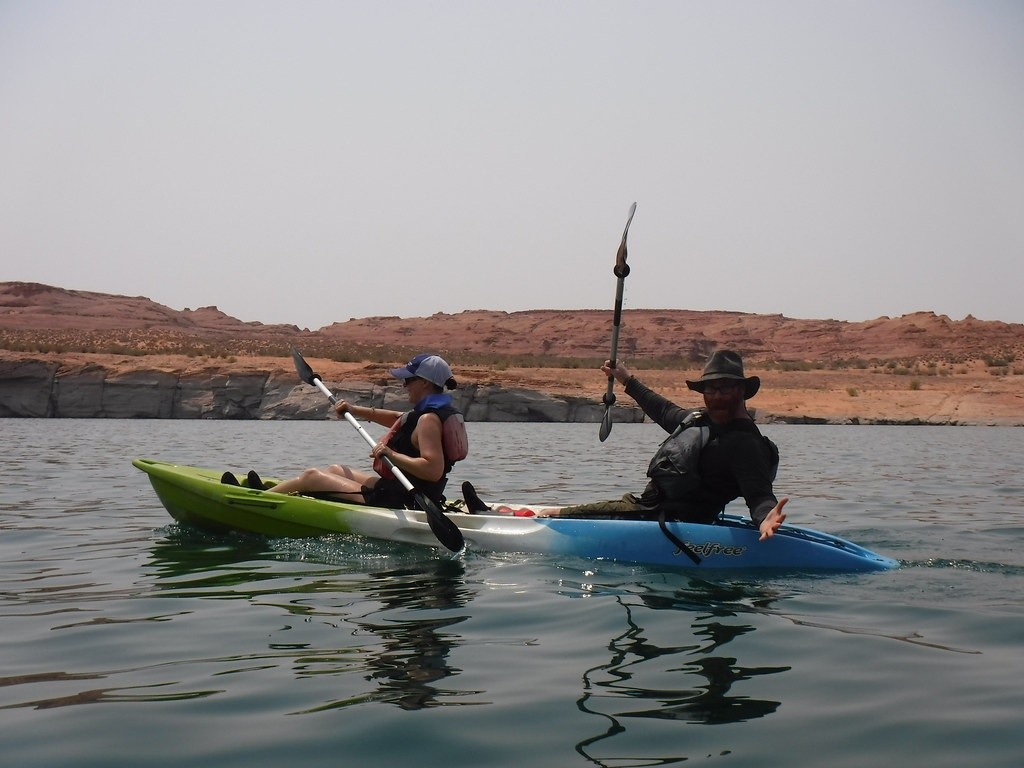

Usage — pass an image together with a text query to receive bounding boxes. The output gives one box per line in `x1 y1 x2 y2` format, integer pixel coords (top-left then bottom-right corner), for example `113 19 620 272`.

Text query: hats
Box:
389 355 454 389
686 350 761 400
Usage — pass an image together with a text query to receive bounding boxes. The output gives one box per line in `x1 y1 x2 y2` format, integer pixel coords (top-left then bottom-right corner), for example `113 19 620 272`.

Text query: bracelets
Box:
623 375 634 386
368 408 374 423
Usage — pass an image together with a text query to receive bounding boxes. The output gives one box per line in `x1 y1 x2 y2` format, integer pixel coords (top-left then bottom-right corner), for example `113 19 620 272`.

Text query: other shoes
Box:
462 481 491 513
221 471 241 486
248 470 267 491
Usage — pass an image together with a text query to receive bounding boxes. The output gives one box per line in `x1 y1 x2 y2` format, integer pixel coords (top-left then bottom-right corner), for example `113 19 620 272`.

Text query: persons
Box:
459 347 791 541
221 353 469 512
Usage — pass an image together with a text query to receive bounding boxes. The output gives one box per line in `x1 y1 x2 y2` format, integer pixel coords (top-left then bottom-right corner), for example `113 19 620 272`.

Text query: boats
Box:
130 456 905 584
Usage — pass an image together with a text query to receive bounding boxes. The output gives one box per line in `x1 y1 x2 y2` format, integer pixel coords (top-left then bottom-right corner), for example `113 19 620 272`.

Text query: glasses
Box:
404 375 422 385
703 382 738 394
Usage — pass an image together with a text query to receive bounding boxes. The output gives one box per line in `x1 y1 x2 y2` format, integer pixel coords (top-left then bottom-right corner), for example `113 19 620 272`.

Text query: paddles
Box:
289 350 469 556
593 200 641 443
